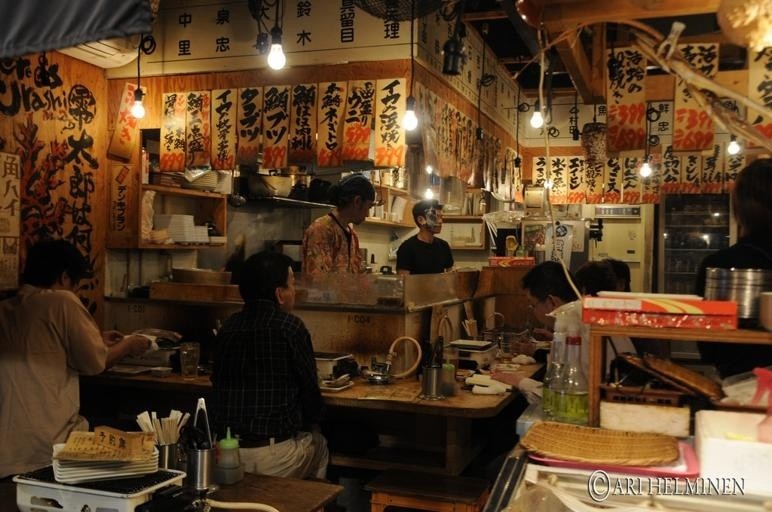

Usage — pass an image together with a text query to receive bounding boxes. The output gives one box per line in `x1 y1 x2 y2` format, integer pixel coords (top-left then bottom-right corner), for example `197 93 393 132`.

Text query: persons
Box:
602 258 661 383
302 172 374 276
694 159 772 377
208 251 328 479
0 242 152 484
490 261 638 404
575 262 637 383
396 200 455 274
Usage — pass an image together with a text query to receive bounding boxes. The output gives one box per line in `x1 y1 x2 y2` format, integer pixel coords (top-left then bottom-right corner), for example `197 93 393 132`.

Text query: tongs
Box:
193 398 213 449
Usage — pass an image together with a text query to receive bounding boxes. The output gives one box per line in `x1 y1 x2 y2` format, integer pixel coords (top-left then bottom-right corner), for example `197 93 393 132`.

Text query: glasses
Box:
524 292 551 311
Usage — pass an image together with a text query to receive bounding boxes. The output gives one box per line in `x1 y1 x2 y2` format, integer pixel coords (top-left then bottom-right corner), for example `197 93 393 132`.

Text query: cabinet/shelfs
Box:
464 187 504 216
414 215 485 252
659 192 737 297
309 182 411 278
110 130 227 249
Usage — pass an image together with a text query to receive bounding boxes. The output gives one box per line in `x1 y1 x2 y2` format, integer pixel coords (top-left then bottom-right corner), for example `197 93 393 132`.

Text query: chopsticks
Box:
136 409 190 445
424 336 444 367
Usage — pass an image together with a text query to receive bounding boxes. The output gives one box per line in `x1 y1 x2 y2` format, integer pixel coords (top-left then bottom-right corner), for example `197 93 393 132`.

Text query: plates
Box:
108 366 150 377
152 170 218 191
52 440 158 484
152 214 210 245
319 380 355 393
210 235 226 247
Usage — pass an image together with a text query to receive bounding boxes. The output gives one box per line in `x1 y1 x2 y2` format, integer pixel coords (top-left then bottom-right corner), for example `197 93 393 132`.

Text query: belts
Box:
237 435 292 449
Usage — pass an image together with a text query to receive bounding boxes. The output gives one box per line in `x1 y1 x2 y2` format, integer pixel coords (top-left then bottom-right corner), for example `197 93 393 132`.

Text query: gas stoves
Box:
444 338 504 371
12 466 281 511
112 336 179 364
314 336 421 384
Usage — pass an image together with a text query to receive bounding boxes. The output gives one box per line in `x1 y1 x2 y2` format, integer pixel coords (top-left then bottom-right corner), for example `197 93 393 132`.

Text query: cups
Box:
179 343 199 381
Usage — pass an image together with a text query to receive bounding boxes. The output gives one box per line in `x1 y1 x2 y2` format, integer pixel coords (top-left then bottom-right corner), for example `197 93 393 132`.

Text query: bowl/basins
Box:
150 367 173 378
368 202 384 220
251 175 295 197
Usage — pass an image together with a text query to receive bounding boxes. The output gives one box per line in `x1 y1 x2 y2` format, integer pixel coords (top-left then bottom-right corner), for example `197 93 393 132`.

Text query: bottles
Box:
219 427 240 469
555 336 587 426
543 334 563 418
442 357 456 397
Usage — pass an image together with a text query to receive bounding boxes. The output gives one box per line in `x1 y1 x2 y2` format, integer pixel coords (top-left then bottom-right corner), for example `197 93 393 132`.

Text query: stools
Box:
365 440 528 512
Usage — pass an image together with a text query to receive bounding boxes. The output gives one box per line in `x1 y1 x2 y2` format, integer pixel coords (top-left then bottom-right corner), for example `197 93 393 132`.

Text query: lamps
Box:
268 27 286 70
530 101 544 128
728 134 740 155
131 88 145 118
640 156 652 178
403 96 418 131
